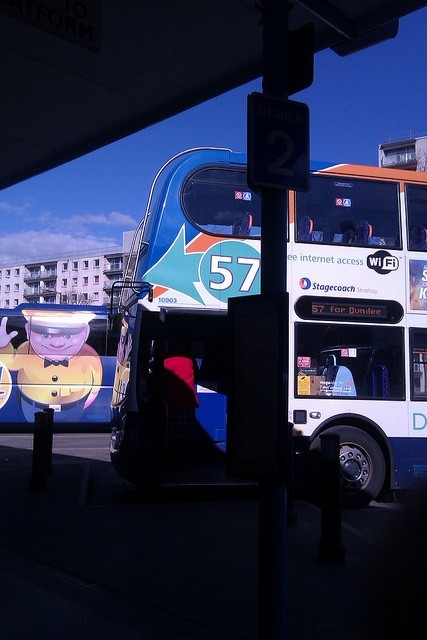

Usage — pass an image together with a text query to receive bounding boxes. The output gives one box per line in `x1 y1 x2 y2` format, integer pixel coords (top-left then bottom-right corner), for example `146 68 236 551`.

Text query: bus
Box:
110 136 427 509
0 303 118 433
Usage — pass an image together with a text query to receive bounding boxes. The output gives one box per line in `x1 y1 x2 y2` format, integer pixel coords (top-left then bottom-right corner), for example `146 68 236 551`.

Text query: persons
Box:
0 309 104 423
